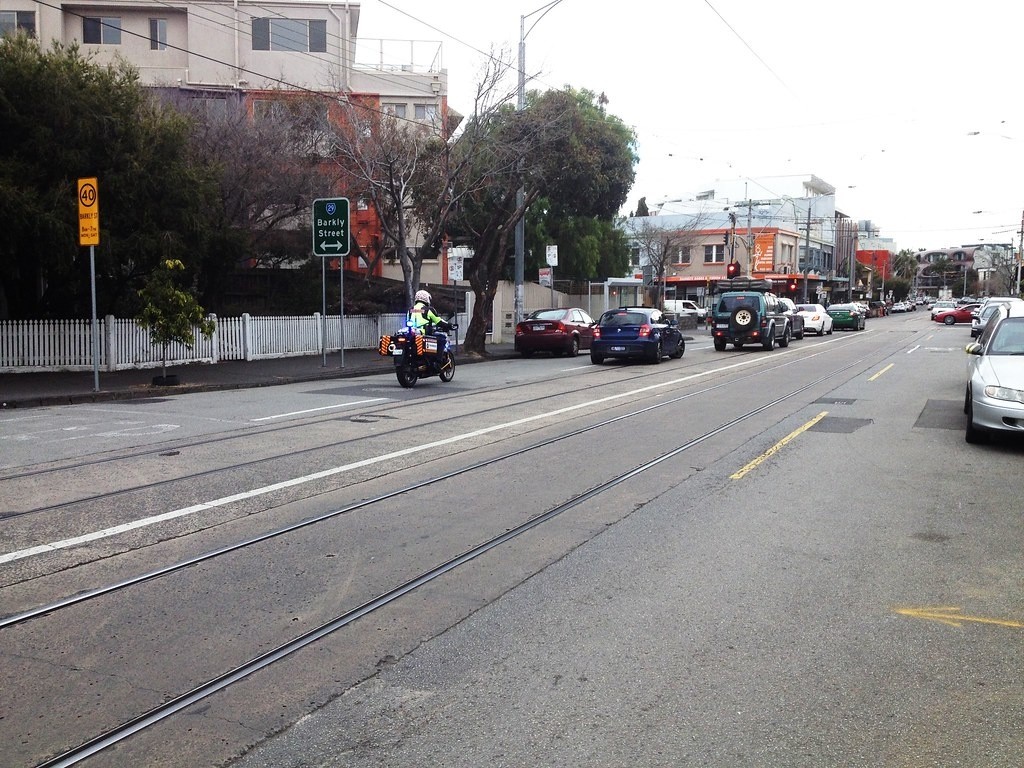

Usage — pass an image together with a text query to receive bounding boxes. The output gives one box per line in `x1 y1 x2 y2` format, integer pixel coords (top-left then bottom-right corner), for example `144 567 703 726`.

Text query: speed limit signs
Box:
77 177 99 245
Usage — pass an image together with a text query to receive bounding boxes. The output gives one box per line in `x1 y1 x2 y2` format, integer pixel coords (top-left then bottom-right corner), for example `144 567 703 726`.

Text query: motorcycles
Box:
382 315 458 385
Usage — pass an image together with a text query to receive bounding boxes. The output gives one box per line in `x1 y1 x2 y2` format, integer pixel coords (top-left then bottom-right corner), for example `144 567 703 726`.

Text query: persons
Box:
407 290 458 373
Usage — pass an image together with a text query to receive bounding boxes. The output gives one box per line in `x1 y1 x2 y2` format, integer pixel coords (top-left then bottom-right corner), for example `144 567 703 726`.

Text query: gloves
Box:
448 322 459 331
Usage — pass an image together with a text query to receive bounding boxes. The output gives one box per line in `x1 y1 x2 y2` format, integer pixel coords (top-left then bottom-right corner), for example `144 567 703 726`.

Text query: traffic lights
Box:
727 265 736 279
788 282 796 294
722 231 729 245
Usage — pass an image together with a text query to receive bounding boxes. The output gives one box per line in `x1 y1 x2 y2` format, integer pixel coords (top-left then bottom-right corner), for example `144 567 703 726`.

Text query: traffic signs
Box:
313 198 349 256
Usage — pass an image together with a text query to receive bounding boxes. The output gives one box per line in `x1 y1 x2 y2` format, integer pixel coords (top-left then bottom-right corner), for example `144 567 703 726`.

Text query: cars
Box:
796 304 834 336
514 308 595 357
904 302 911 312
591 309 685 363
968 300 976 304
931 301 955 320
966 304 1024 442
928 301 937 310
891 303 906 313
956 297 968 304
778 297 804 340
827 304 865 330
909 300 916 311
971 296 1024 337
923 297 936 304
934 305 982 324
916 297 923 305
855 301 891 318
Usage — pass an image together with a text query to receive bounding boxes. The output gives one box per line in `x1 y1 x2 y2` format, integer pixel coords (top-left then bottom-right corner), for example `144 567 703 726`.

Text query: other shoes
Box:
430 362 441 373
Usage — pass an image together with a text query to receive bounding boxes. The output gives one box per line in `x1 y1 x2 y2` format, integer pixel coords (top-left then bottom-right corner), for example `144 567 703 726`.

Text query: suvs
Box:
711 293 790 351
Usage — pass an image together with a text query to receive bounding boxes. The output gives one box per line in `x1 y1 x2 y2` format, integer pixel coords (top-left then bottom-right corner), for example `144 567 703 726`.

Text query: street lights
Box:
801 185 854 302
972 210 1024 296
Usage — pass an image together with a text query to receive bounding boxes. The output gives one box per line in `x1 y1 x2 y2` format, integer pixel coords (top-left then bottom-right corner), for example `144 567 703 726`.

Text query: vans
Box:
664 300 707 323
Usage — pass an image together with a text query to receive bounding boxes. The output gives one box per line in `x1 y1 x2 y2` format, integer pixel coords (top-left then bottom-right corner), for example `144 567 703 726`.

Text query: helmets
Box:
415 290 432 307
